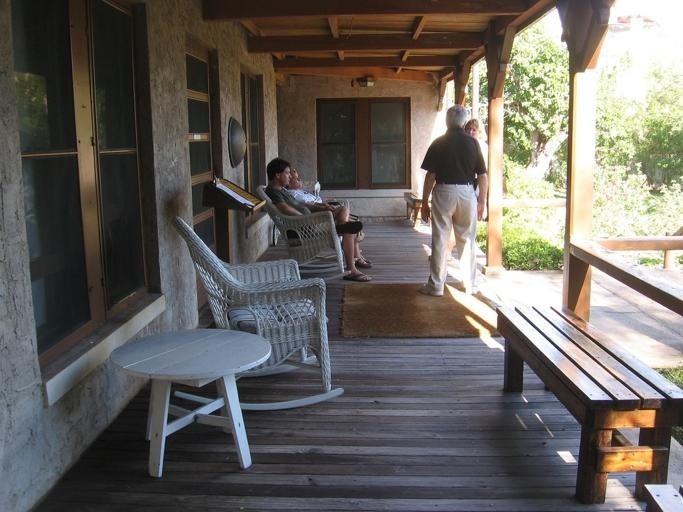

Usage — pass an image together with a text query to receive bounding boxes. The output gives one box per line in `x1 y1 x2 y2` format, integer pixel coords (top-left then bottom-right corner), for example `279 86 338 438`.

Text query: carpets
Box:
337 282 509 339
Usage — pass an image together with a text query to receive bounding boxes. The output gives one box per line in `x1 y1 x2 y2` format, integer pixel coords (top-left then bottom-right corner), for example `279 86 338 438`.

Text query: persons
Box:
285 167 371 268
426 118 489 265
265 159 373 282
417 105 490 297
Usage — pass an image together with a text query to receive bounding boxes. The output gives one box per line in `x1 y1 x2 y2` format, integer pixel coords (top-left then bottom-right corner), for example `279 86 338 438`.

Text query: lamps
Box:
350 75 375 88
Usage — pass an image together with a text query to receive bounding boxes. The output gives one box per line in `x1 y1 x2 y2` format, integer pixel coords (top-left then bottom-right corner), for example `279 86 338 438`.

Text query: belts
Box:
436 180 474 185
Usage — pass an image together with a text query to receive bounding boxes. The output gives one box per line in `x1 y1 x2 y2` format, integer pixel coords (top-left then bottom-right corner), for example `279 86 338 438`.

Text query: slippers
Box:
342 273 372 282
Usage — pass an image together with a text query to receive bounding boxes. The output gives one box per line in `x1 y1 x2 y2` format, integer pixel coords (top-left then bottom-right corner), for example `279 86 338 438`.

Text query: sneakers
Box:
418 283 444 297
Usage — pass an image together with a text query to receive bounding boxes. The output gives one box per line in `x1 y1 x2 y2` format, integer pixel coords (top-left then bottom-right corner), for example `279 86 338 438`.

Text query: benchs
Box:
403 192 430 228
641 485 683 511
496 306 682 505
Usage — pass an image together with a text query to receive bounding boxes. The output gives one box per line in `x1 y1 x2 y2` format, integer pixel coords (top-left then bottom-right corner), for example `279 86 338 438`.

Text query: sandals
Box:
355 258 372 267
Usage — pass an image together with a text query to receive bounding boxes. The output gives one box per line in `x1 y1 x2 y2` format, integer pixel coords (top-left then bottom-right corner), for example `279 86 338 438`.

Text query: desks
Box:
110 328 271 479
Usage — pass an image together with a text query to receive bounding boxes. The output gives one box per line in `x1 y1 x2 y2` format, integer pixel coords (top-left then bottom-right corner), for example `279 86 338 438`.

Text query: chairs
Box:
170 215 344 412
324 199 350 208
256 183 350 282
